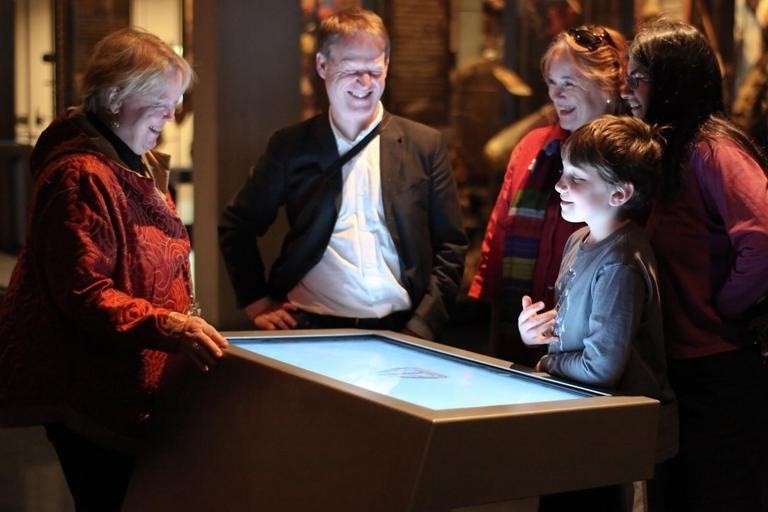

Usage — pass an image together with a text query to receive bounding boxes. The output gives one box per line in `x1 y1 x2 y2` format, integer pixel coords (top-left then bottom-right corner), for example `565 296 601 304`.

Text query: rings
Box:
190 341 203 352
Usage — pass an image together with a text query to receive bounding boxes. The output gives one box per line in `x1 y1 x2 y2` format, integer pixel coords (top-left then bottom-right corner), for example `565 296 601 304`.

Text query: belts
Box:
301 309 411 329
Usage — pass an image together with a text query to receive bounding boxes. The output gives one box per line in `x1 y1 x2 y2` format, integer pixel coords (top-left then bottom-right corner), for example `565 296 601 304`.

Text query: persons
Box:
218 8 474 343
464 19 632 368
622 13 767 512
512 114 684 512
0 25 231 510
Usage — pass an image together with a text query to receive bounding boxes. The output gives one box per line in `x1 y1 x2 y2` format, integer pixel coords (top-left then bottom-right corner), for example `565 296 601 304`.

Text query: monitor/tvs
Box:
225 333 611 412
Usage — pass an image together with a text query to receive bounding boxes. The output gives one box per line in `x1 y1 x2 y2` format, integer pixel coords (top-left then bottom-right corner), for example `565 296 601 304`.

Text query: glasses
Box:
621 70 649 90
564 26 609 52
